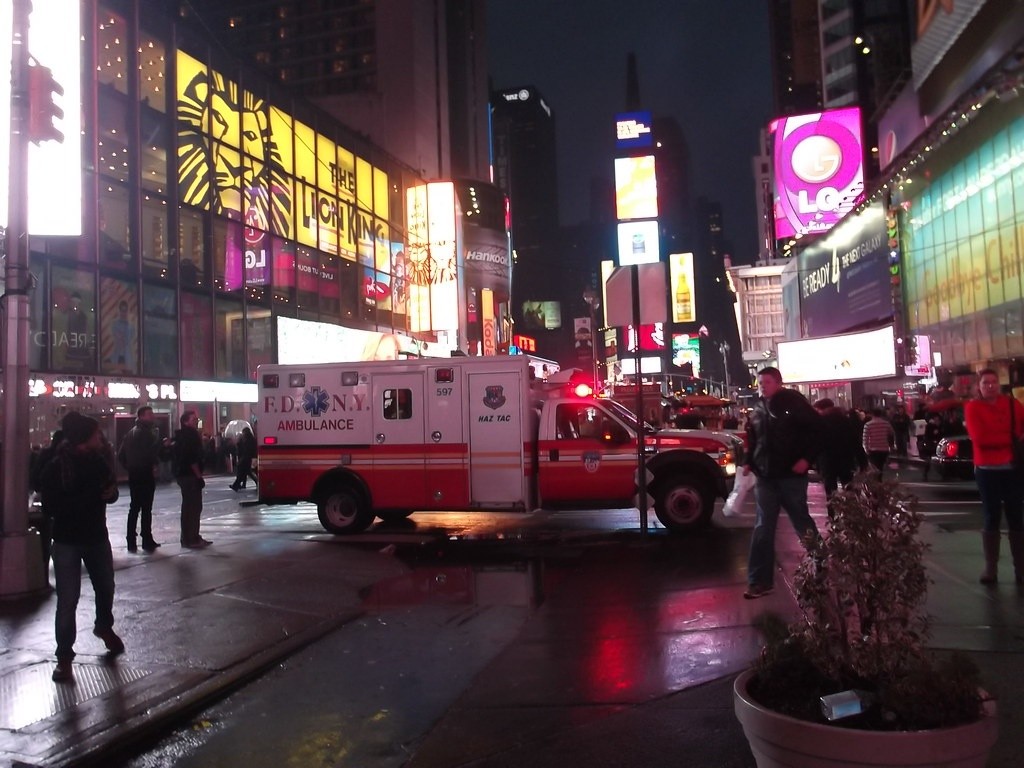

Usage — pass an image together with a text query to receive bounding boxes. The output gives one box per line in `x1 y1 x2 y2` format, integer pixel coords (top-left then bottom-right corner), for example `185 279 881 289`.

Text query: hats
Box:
814 398 834 409
63 411 98 445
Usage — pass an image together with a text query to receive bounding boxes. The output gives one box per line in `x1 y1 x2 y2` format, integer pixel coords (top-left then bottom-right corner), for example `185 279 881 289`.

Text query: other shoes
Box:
128 539 136 550
229 484 239 493
744 584 775 598
52 661 72 679
181 534 213 548
143 536 161 549
93 626 124 651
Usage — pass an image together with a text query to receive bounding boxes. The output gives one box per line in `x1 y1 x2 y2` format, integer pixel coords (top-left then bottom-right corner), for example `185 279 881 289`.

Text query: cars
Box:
930 437 981 502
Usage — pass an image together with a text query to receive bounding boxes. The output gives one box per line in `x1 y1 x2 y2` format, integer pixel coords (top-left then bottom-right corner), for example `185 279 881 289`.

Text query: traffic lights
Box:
30 66 64 147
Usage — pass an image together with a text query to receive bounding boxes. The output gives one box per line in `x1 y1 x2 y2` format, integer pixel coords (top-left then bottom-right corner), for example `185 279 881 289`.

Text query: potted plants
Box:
732 467 998 768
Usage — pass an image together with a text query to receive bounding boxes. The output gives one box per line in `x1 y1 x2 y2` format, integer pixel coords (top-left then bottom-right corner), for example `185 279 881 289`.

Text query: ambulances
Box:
257 346 746 537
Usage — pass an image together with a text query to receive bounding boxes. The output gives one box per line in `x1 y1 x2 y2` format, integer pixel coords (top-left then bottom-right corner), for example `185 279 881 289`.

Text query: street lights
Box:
584 290 600 398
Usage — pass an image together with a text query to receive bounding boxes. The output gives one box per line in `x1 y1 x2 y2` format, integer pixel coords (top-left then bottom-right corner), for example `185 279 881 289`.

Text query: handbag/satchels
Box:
723 466 757 518
1010 397 1024 470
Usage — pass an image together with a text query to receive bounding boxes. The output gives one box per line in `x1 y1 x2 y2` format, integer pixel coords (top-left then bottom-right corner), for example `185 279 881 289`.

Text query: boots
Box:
1009 531 1024 582
980 529 1000 583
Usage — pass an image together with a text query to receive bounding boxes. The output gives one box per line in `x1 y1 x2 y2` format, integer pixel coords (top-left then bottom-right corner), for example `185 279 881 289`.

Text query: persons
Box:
175 411 213 549
723 413 738 429
849 406 964 481
30 411 125 686
117 406 174 553
965 369 1024 590
740 367 829 598
814 398 855 519
154 426 258 492
